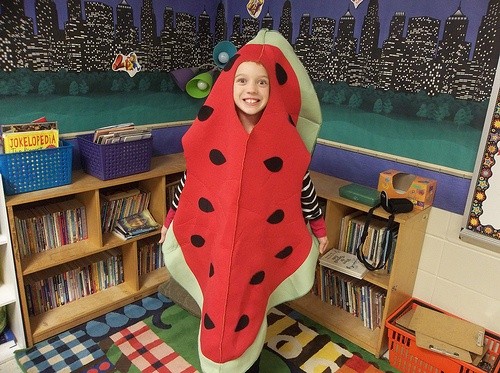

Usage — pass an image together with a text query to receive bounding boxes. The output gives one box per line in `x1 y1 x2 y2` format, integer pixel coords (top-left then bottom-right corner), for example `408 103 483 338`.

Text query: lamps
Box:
171 41 237 98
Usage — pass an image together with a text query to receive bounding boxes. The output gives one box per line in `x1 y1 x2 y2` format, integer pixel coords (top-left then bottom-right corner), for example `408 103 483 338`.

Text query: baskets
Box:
385 298 499 373
76 131 153 182
0 137 75 197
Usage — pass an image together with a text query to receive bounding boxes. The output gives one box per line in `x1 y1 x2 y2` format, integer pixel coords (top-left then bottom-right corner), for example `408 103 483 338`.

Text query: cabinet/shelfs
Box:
0 152 432 365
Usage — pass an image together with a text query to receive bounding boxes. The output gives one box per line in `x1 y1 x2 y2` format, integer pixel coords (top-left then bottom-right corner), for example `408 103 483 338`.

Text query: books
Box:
0 117 398 352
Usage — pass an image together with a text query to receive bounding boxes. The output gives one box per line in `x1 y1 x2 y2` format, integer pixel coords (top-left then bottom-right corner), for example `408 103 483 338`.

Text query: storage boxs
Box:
76 134 153 181
0 139 75 196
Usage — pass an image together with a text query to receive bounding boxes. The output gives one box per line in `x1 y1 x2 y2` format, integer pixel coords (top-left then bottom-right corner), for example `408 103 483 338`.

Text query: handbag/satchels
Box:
381 189 414 214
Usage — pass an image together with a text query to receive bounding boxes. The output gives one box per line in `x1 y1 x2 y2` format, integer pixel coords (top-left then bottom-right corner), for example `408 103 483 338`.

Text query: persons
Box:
159 28 329 373
